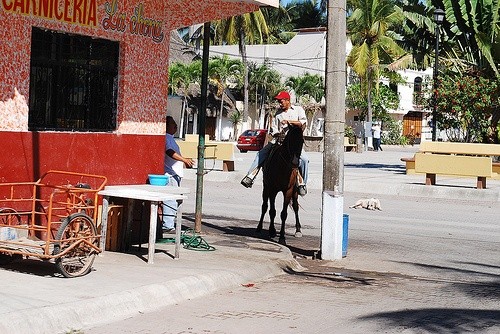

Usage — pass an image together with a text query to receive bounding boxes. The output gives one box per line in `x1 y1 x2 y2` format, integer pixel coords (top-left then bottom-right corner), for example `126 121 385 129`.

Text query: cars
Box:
236 129 268 152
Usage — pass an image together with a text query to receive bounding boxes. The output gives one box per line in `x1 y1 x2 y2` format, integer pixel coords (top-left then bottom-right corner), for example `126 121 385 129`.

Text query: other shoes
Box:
243 177 253 188
297 185 306 195
162 228 176 234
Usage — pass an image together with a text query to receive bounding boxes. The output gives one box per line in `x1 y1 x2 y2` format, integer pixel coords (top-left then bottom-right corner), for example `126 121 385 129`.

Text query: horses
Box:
256 119 306 246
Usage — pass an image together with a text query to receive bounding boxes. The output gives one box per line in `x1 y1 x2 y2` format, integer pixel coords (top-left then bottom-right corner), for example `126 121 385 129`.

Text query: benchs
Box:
414 141 500 189
176 141 234 171
343 137 357 152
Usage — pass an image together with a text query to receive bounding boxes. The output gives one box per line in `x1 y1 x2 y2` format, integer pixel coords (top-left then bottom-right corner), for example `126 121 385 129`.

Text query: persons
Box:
240 91 306 196
370 122 383 151
161 116 194 229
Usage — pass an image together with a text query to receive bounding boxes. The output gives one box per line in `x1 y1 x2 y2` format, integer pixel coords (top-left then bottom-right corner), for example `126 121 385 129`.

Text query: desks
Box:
97 184 191 264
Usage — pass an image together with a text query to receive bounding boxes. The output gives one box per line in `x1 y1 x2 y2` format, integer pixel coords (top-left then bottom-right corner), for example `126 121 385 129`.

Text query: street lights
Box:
431 5 446 141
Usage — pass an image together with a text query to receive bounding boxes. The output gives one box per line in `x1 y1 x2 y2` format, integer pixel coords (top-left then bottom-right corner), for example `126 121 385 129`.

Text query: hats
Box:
274 91 290 100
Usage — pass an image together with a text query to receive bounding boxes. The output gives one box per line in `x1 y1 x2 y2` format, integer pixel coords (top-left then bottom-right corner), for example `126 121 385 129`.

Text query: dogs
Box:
348 197 383 211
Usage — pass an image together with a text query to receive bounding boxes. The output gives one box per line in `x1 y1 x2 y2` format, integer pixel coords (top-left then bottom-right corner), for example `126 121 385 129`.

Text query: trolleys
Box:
0 170 109 279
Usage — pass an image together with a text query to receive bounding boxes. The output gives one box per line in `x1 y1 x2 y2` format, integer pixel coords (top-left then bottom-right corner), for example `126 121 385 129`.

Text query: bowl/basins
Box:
148 174 169 186
